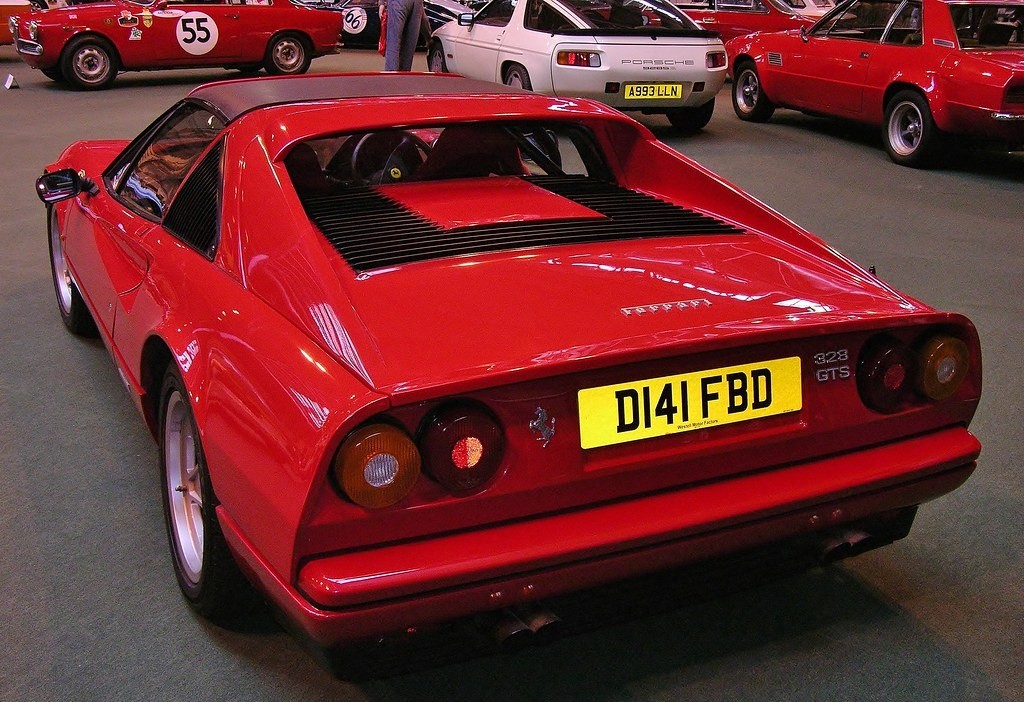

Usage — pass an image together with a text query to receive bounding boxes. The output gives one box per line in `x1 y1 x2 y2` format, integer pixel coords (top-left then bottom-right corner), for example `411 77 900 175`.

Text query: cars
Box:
426 0 728 131
35 73 982 685
724 0 1023 166
0 0 858 91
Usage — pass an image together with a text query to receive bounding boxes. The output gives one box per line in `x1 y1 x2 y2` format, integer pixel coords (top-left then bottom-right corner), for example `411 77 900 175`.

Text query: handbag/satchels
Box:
420 11 432 43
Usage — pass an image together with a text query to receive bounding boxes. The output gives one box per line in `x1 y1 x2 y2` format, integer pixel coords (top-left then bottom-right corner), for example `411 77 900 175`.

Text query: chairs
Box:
609 5 643 27
284 143 330 191
538 5 568 30
413 128 531 181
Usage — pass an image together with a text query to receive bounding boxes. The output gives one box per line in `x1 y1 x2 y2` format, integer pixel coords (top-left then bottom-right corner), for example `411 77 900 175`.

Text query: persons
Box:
379 0 434 71
